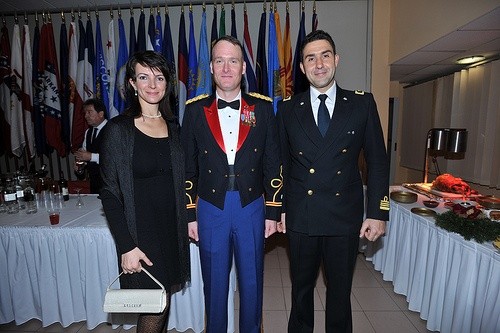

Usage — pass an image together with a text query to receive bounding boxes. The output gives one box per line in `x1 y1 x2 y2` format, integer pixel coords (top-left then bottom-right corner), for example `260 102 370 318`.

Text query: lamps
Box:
424 127 469 183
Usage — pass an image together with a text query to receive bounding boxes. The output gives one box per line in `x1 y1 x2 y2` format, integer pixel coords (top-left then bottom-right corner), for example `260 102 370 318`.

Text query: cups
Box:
48 208 59 225
35 190 54 209
53 193 65 208
49 184 60 194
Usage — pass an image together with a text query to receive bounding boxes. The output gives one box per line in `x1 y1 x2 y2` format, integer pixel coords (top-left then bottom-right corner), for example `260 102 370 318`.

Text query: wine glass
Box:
74 187 84 208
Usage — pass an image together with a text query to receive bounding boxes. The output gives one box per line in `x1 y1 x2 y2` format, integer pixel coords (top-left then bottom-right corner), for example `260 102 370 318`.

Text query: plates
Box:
411 208 436 216
390 191 418 203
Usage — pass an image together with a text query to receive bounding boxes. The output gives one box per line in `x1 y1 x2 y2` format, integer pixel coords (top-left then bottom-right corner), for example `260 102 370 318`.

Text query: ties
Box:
317 94 331 139
92 128 98 144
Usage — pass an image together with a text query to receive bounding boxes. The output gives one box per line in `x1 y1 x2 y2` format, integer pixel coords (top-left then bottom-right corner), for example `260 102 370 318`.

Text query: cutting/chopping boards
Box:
416 182 482 198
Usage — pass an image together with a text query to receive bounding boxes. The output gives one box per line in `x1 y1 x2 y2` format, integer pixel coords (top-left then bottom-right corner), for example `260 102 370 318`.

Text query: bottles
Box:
3 180 19 214
13 179 25 211
24 184 37 214
0 179 7 213
58 171 69 201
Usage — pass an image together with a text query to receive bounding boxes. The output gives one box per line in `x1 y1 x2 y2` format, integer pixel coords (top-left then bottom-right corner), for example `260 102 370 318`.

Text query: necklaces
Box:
142 112 161 119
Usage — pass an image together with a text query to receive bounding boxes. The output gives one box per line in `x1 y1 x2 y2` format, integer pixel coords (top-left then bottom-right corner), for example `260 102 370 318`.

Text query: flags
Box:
0 19 117 158
112 10 212 115
212 8 318 114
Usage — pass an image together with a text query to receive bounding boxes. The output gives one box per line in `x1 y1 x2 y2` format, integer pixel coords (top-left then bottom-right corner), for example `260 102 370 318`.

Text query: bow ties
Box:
218 98 240 110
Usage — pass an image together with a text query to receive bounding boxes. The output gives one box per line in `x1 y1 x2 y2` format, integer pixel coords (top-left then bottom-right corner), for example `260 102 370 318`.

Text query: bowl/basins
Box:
423 201 439 208
490 211 500 220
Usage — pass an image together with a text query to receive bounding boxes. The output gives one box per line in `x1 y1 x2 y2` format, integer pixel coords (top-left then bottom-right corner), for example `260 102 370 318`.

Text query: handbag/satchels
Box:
103 267 168 314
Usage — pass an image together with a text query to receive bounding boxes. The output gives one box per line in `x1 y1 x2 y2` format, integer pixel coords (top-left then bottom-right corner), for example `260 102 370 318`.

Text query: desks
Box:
0 194 236 333
361 187 500 333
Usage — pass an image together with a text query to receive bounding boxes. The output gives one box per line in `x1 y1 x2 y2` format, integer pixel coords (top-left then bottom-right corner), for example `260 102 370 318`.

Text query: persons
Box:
276 29 389 333
98 50 191 333
181 34 282 333
73 98 108 194
